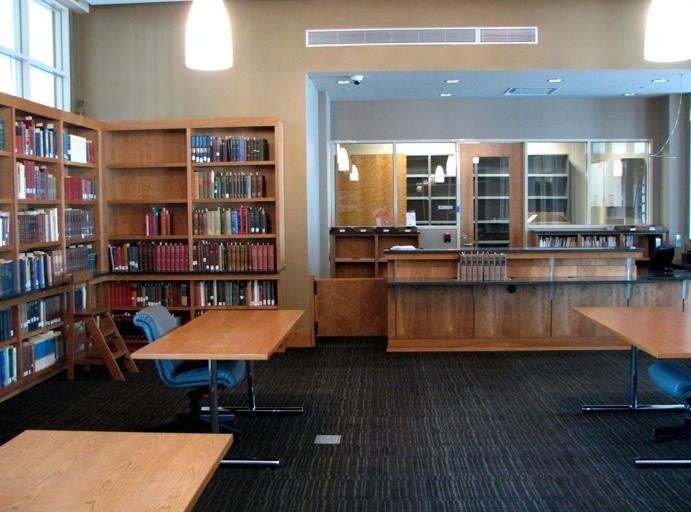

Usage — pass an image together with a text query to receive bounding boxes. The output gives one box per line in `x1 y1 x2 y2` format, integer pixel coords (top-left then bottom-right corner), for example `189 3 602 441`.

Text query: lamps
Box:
435 154 457 183
337 144 358 181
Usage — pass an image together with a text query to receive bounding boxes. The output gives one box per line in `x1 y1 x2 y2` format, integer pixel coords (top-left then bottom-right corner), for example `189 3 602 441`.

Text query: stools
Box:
646 360 691 440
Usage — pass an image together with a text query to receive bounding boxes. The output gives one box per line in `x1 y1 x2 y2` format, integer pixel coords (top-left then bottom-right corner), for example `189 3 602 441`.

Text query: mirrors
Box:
589 139 652 226
526 139 589 226
395 139 460 229
332 139 394 229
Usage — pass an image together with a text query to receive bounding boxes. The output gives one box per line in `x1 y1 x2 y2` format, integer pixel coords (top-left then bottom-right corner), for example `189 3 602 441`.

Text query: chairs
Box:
131 302 247 438
649 247 674 272
65 271 138 384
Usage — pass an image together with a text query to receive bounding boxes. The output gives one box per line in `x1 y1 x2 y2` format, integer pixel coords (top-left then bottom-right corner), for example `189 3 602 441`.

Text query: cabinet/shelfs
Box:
384 246 691 352
1 94 64 401
528 226 669 250
332 232 420 278
102 121 188 359
61 110 104 383
190 119 286 352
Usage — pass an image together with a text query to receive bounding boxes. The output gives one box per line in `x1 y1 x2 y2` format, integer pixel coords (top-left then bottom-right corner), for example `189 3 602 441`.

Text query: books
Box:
457 250 507 280
538 234 639 248
106 204 190 341
0 112 100 392
189 132 277 319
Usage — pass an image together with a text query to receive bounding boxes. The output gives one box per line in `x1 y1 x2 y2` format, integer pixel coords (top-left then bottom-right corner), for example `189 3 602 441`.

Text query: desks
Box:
573 306 691 465
131 305 305 466
1 429 234 512
